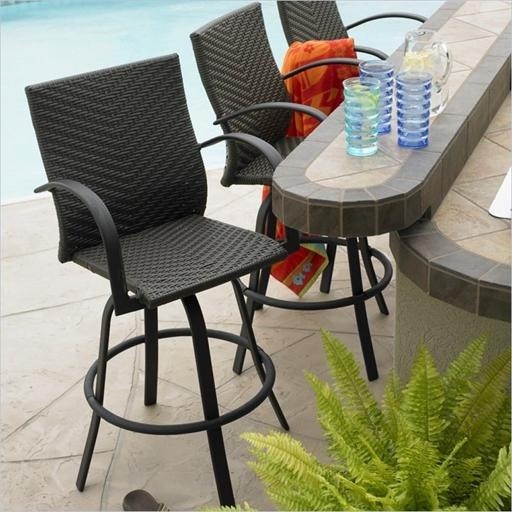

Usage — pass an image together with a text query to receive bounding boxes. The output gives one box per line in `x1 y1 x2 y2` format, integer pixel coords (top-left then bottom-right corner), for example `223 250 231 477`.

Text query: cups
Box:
403 29 451 116
342 76 382 158
395 71 432 148
358 60 393 135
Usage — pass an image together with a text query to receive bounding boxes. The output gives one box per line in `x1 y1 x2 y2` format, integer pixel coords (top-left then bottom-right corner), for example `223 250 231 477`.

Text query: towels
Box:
249 36 360 299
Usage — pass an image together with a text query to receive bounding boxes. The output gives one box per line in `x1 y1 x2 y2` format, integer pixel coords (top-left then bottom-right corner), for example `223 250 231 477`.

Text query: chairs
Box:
276 1 435 92
190 0 395 385
21 54 291 506
